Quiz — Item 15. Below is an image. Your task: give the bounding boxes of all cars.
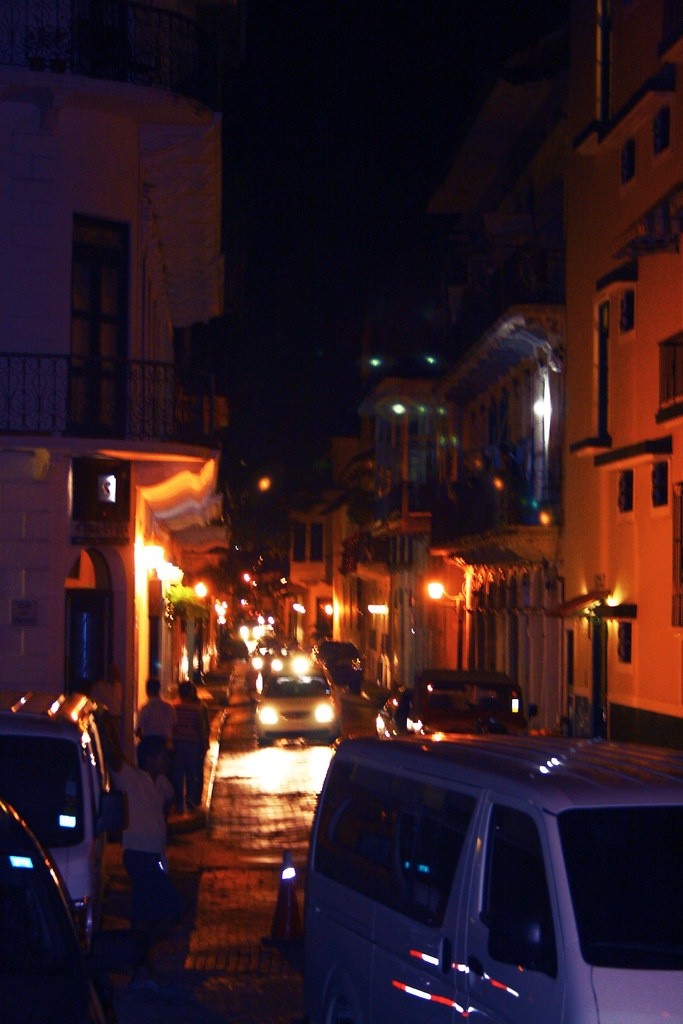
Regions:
[407,668,527,732]
[257,671,340,745]
[313,640,364,693]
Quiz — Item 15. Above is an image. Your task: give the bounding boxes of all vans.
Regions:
[1,691,114,956]
[0,799,120,1024]
[300,730,683,1023]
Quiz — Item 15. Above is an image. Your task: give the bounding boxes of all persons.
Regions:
[141,677,210,814]
[104,737,183,980]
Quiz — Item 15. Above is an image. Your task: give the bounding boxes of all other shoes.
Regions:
[188,801,197,814]
[172,800,184,816]
